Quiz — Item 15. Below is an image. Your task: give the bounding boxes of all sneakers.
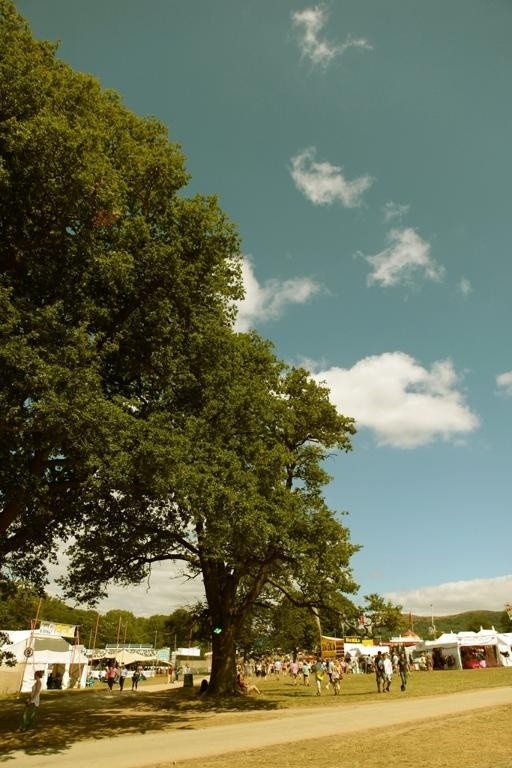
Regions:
[378,684,405,693]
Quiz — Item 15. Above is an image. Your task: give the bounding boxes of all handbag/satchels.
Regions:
[317,673,323,681]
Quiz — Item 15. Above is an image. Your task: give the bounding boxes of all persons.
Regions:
[87,662,191,691]
[53,672,62,688]
[237,651,433,696]
[47,672,52,688]
[16,670,45,732]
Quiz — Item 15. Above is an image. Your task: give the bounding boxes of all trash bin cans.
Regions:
[184,674,193,687]
